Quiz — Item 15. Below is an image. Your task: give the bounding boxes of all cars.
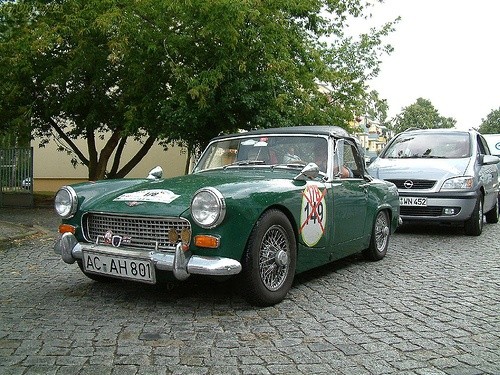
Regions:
[366,127,500,236]
[54,125,401,305]
